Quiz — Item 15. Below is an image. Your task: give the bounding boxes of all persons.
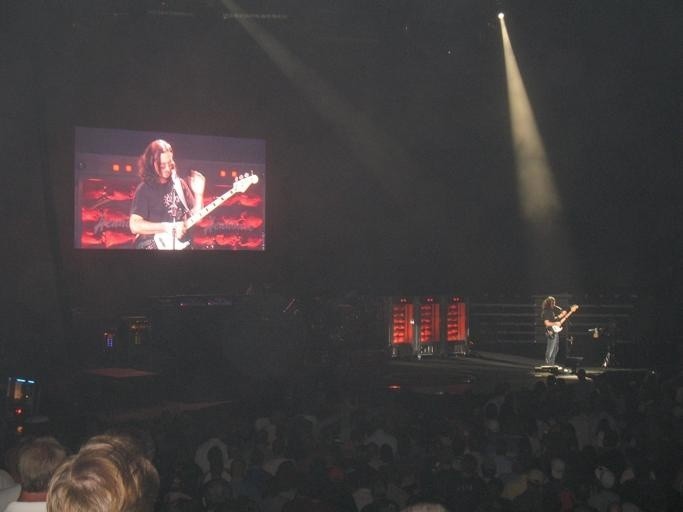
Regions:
[540,295,567,366]
[592,318,618,369]
[128,138,207,251]
[0,365,683,511]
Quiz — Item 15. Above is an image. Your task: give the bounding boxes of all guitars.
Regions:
[543,305,577,339]
[134,170,258,251]
